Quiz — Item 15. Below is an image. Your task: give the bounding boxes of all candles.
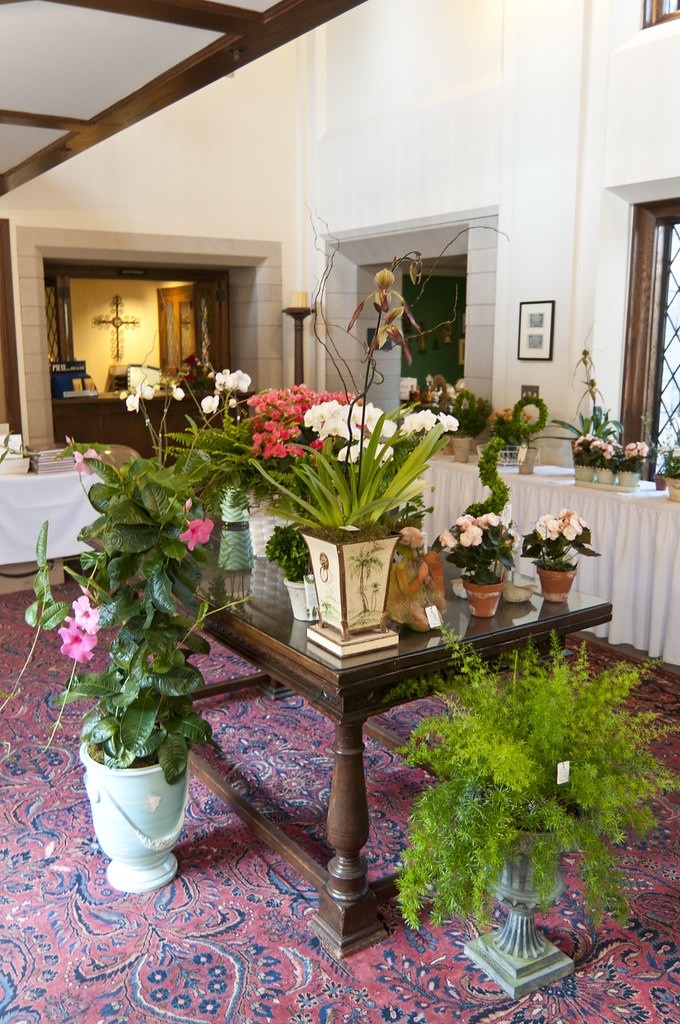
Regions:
[289,290,308,308]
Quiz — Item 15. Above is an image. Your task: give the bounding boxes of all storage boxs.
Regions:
[0,556,65,596]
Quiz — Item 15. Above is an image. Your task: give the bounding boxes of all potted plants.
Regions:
[511,396,548,474]
[264,525,319,622]
[447,390,493,463]
[393,628,680,1001]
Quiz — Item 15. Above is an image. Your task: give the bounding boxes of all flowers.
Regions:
[0,219,680,785]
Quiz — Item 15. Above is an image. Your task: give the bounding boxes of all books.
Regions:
[25,441,77,477]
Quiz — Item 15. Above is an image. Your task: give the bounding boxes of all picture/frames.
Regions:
[518,300,555,361]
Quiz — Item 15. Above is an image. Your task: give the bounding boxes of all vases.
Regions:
[617,471,640,487]
[247,494,294,558]
[537,567,576,603]
[462,577,504,617]
[298,529,404,660]
[219,485,254,570]
[575,465,595,482]
[80,744,189,893]
[595,467,615,485]
[653,474,668,491]
[665,477,680,502]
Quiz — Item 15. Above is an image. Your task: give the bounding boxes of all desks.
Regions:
[420,454,680,668]
[51,388,256,468]
[0,474,105,565]
[84,501,613,961]
